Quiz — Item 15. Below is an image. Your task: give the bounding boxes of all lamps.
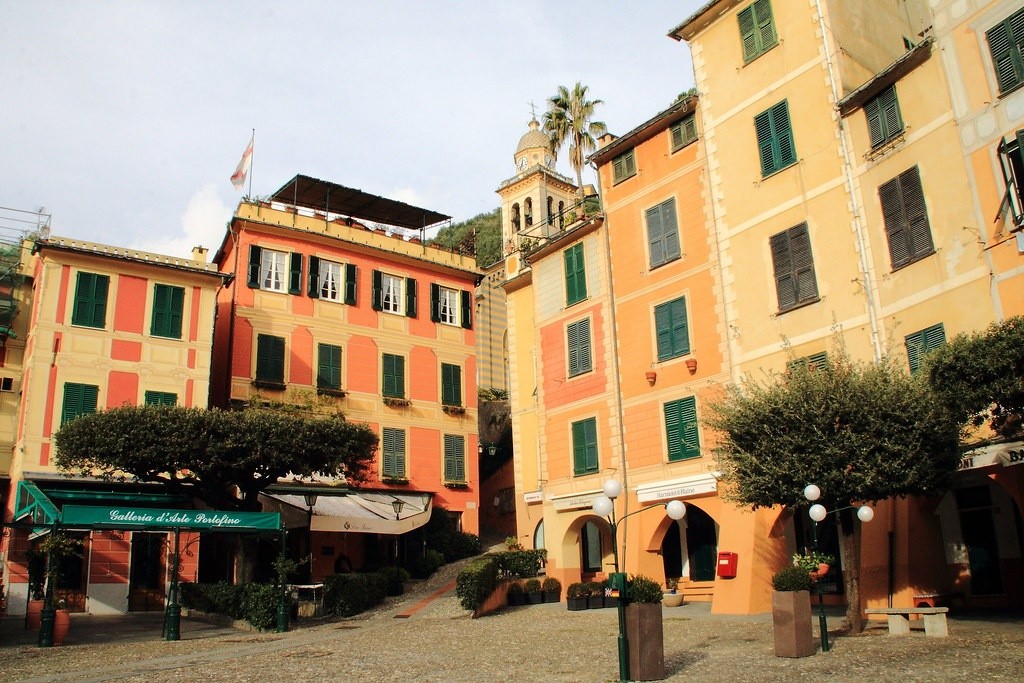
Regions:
[488,442,497,455]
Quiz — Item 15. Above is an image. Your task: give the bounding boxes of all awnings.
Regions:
[262,486,434,536]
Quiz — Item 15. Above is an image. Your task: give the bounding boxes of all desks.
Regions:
[913,593,942,620]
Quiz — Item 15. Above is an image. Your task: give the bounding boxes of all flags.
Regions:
[229,138,253,192]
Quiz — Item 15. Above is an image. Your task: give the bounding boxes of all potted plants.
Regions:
[772,566,815,658]
[23,548,46,634]
[506,577,684,611]
[623,572,666,681]
[40,553,85,646]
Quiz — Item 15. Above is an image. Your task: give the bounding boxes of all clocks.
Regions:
[517,156,528,171]
[543,154,556,169]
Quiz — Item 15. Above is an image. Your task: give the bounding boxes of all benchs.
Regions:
[865,607,949,637]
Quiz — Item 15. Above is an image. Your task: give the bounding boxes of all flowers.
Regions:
[791,552,835,582]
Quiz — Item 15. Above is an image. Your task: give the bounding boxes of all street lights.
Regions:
[804,484,875,653]
[591,480,686,683]
